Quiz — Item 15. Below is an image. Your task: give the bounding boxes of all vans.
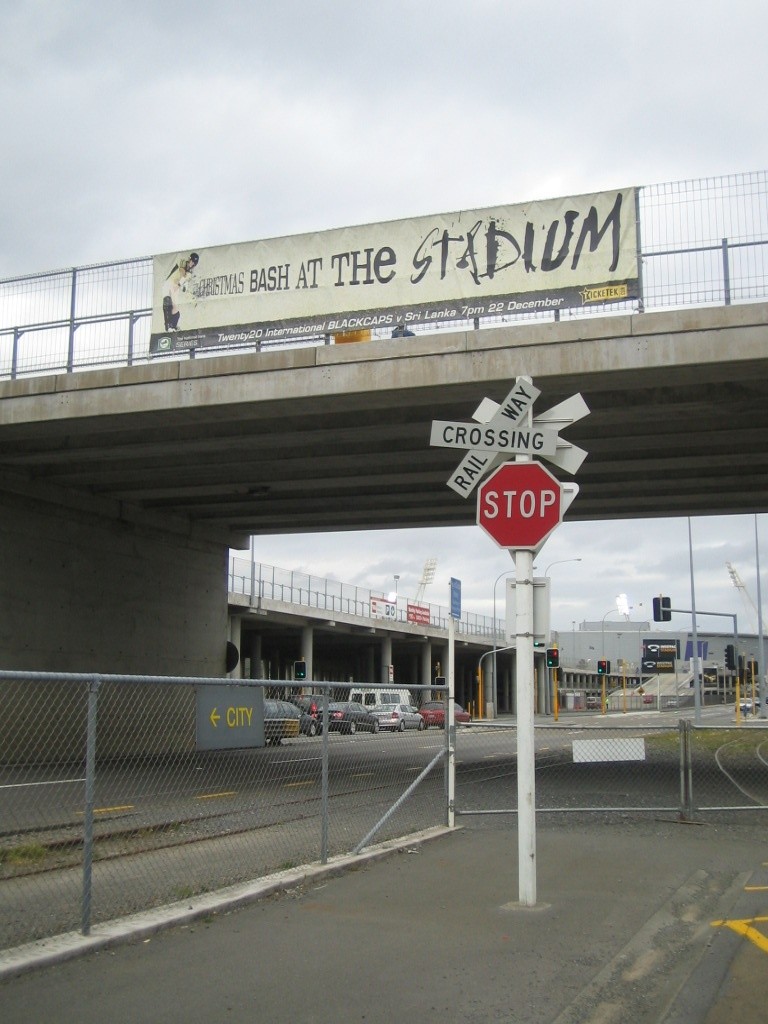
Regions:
[348,687,419,712]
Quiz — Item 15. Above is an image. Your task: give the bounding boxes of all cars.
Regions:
[287,694,380,735]
[419,701,472,729]
[264,698,318,741]
[735,697,760,712]
[369,703,425,732]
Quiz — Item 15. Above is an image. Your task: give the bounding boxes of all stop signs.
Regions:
[475,461,564,549]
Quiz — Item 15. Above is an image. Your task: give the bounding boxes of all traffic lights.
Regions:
[534,642,545,647]
[597,660,607,674]
[546,648,560,668]
[723,644,734,670]
[294,661,307,679]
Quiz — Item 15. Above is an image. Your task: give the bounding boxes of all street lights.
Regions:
[493,567,537,719]
[545,558,583,577]
